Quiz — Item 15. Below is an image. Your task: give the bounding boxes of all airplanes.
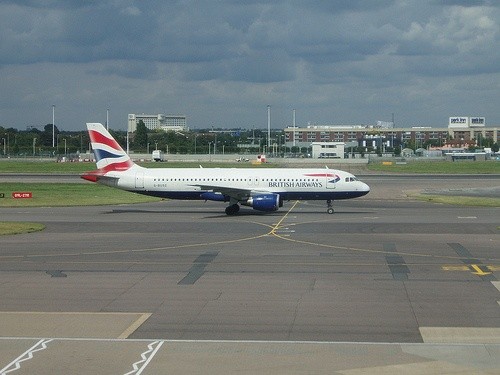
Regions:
[79,121,370,217]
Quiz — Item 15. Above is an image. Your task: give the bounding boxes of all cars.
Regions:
[235,156,249,162]
